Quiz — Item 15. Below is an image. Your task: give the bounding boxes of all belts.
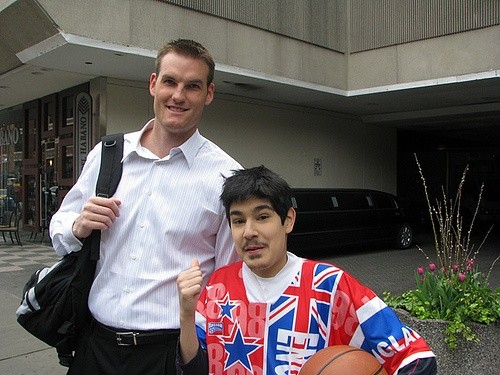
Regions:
[91,317,180,346]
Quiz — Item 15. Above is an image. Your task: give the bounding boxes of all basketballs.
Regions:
[299,345,388,375]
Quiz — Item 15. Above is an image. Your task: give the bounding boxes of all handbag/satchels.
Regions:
[16,248,100,366]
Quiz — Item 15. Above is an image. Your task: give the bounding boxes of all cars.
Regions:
[285,188,426,252]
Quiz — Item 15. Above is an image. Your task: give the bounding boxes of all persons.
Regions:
[48,37,246,375]
[176,166,438,375]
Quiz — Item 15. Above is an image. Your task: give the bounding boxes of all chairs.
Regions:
[0,210,51,246]
